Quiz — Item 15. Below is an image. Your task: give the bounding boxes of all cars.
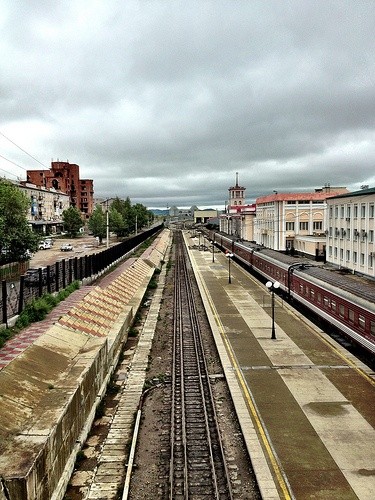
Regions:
[37,241,49,251]
[44,239,53,245]
[60,244,74,252]
[24,266,55,286]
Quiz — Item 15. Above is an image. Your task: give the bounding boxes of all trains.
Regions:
[197,225,375,356]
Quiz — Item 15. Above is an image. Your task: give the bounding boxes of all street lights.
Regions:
[225,253,234,284]
[266,281,280,339]
[197,232,202,251]
[210,240,217,262]
